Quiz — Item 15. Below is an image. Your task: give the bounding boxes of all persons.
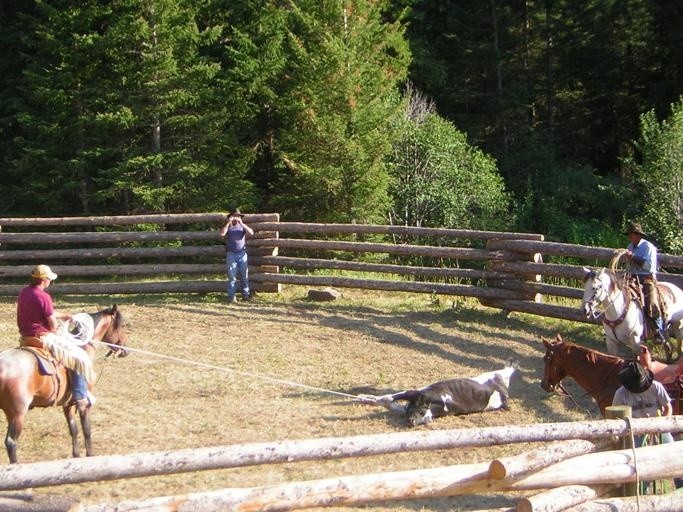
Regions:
[218,207,254,304]
[612,362,683,496]
[17,264,91,412]
[622,223,667,344]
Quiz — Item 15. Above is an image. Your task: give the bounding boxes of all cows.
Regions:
[356,354,520,428]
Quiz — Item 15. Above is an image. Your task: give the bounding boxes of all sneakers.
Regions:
[77,397,95,408]
[228,296,238,302]
[241,293,251,300]
[654,336,665,345]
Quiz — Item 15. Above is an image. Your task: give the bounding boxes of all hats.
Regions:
[228,209,244,218]
[31,264,57,280]
[622,223,647,235]
[618,361,654,394]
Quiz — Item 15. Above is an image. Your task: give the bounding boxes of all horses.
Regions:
[580,265,683,362]
[0,303,130,464]
[540,331,683,420]
